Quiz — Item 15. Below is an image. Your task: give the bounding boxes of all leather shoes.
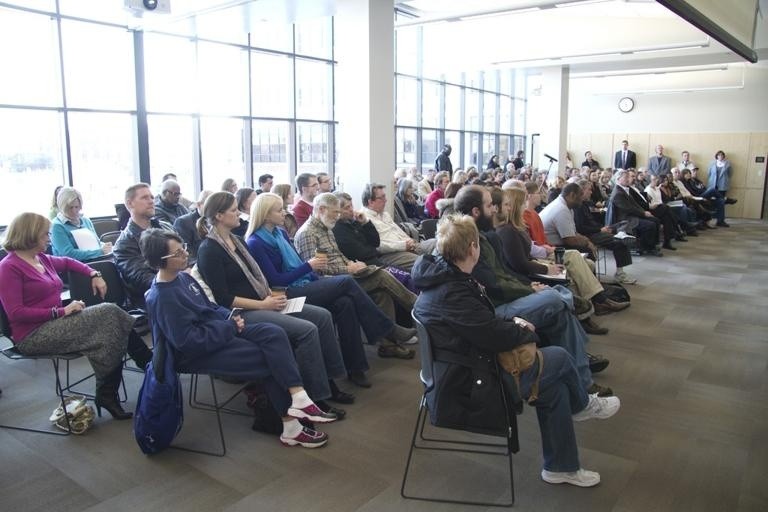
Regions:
[683,222,699,231]
[662,239,676,250]
[329,379,356,404]
[724,197,737,204]
[647,247,663,257]
[316,400,345,421]
[580,318,609,334]
[592,292,630,316]
[717,220,730,227]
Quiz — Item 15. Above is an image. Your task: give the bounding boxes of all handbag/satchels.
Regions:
[50,396,96,435]
[244,377,292,432]
[498,321,543,405]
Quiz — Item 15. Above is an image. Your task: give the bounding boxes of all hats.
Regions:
[688,163,699,171]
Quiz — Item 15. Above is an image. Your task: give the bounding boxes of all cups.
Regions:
[270,286,286,309]
[554,247,565,263]
[315,248,328,270]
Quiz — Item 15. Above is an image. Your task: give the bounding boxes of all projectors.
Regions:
[123,0,170,15]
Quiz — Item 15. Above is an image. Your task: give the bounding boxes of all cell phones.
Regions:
[227,308,242,320]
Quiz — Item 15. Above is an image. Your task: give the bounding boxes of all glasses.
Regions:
[160,243,187,259]
[320,179,331,183]
[324,206,341,216]
[166,190,182,197]
[529,192,541,195]
[602,177,609,178]
[43,232,52,238]
[375,194,386,200]
[303,183,319,187]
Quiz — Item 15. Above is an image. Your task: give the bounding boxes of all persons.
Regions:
[394,168,407,181]
[397,178,419,220]
[418,169,436,199]
[676,152,695,171]
[574,180,637,284]
[507,189,571,289]
[522,182,596,275]
[453,163,548,205]
[140,229,337,448]
[502,180,630,335]
[615,140,636,169]
[162,174,195,208]
[393,178,418,229]
[173,190,218,255]
[503,155,515,172]
[0,212,154,419]
[331,191,383,267]
[486,187,609,374]
[581,151,599,171]
[436,183,463,217]
[408,168,419,179]
[453,185,610,396]
[295,193,418,360]
[487,155,500,169]
[273,184,298,238]
[604,168,738,257]
[154,180,191,229]
[547,166,617,212]
[513,151,524,170]
[411,212,621,488]
[359,185,437,272]
[50,187,112,261]
[566,152,573,169]
[707,150,732,195]
[293,173,320,228]
[538,184,597,262]
[49,186,62,220]
[317,172,331,192]
[236,189,258,235]
[256,174,273,194]
[424,171,450,218]
[434,145,452,181]
[112,182,173,306]
[244,193,417,387]
[222,178,238,193]
[196,192,355,422]
[646,145,672,176]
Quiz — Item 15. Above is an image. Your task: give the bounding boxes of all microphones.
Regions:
[543,154,558,163]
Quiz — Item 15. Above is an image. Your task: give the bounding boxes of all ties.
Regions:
[685,161,687,167]
[622,152,625,170]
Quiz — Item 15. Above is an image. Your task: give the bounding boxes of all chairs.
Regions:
[401,308,513,508]
[93,220,121,246]
[143,290,257,457]
[0,301,127,436]
[65,261,147,403]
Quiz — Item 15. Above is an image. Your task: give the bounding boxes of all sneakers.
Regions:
[572,395,620,422]
[587,382,613,397]
[378,344,416,360]
[396,336,418,344]
[613,272,637,285]
[586,351,609,374]
[541,469,600,487]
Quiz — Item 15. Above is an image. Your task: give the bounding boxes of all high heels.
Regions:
[674,225,689,242]
[135,344,152,373]
[95,385,133,420]
[385,324,417,345]
[347,370,371,388]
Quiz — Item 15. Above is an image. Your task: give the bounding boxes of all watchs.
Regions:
[90,272,103,277]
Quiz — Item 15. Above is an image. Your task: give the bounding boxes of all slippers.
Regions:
[280,426,329,448]
[287,404,338,424]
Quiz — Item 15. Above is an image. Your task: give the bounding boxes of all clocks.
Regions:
[618,97,634,113]
